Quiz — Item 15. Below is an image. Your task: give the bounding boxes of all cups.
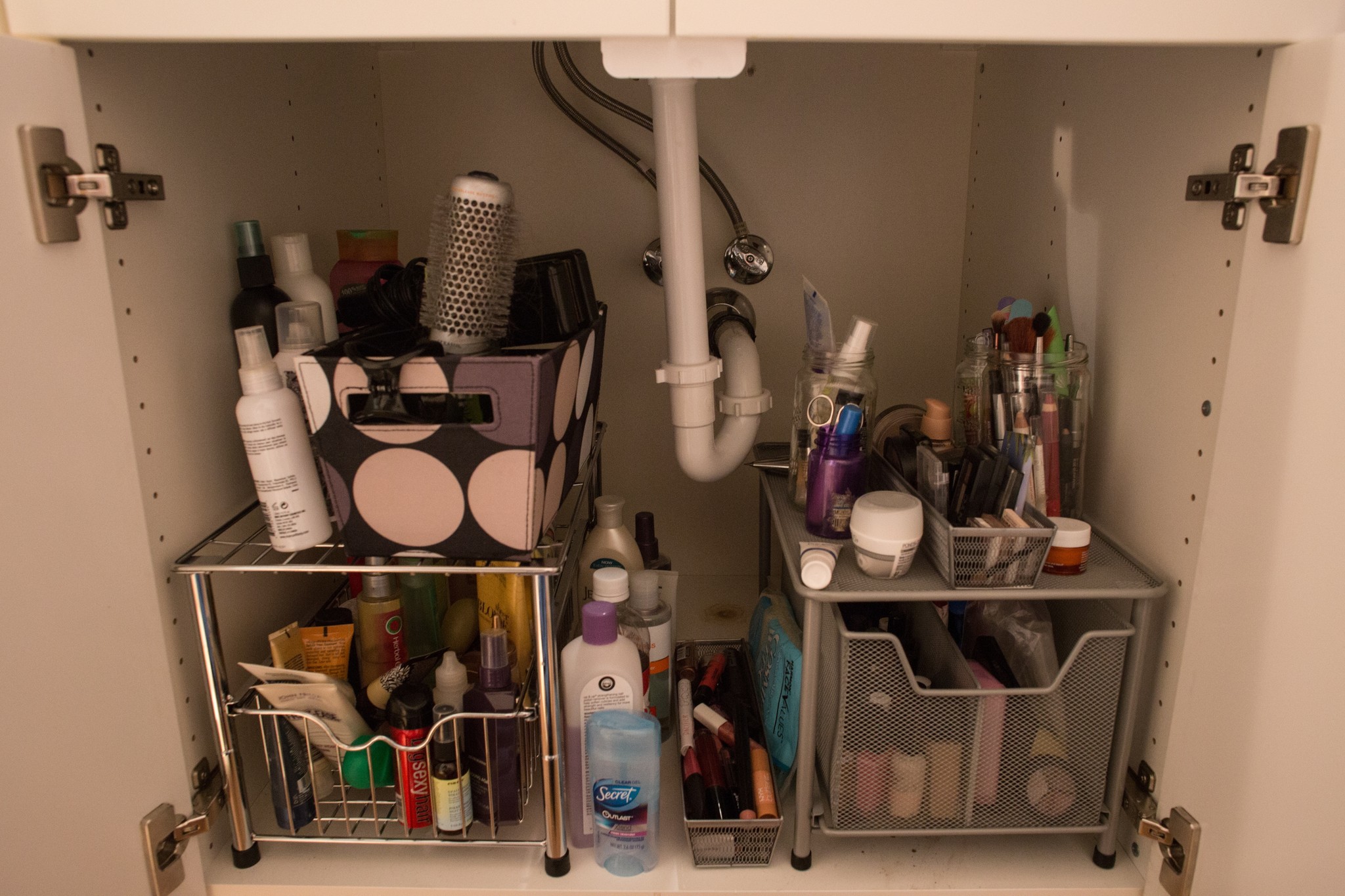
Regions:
[794,341,877,516]
[978,340,1090,523]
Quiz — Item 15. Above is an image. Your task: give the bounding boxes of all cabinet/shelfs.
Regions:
[0,0,1345,894]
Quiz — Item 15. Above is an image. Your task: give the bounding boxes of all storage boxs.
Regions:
[296,299,610,562]
